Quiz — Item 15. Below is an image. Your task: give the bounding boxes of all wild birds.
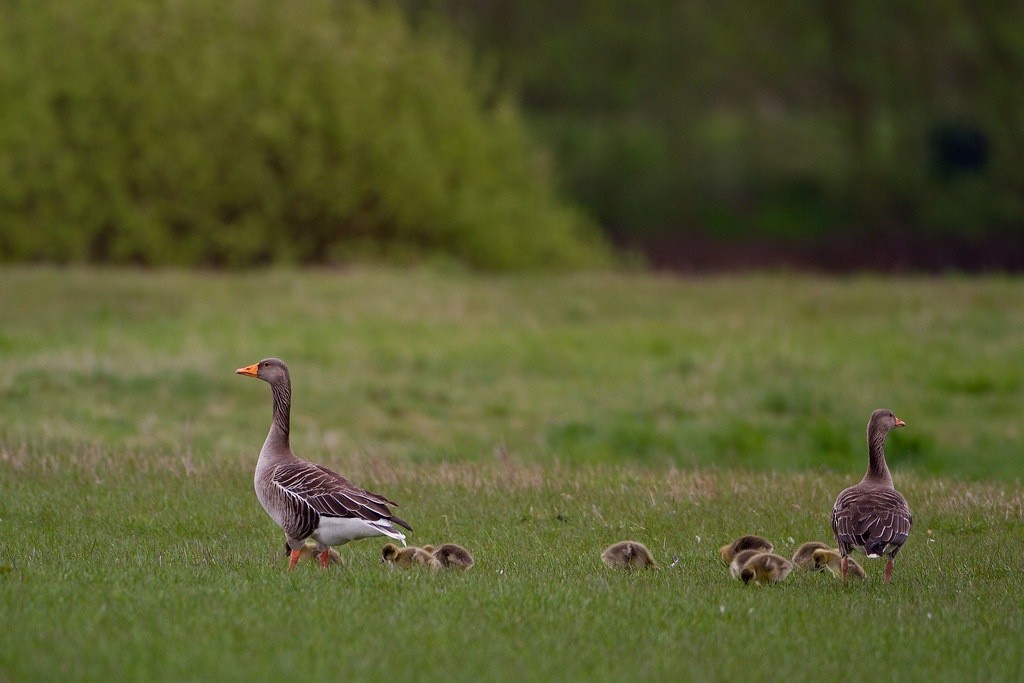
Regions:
[237,358,475,574]
[718,534,866,588]
[830,408,913,591]
[600,541,659,585]
